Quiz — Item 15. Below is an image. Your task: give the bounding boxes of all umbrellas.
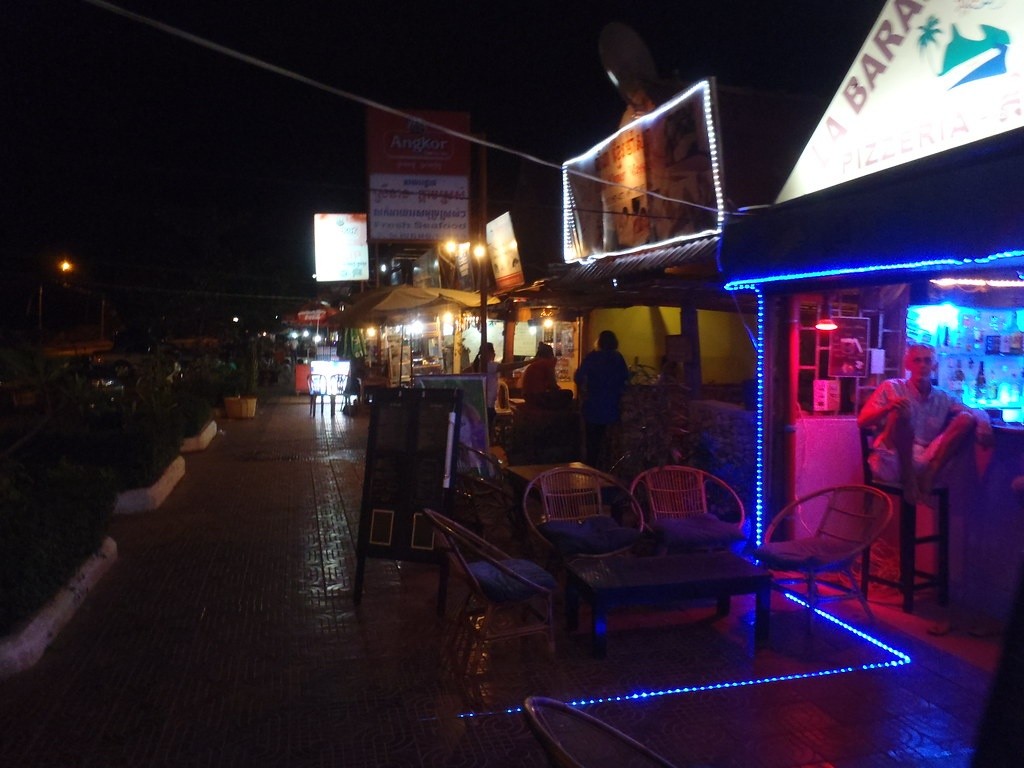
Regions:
[324,284,500,388]
[281,301,341,337]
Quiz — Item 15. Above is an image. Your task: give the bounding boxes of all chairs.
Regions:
[522,467,646,571]
[521,696,677,768]
[752,485,894,637]
[306,374,360,418]
[424,509,559,680]
[629,466,746,554]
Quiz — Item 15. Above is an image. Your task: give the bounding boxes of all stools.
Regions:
[860,481,951,613]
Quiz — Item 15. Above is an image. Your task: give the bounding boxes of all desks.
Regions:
[565,547,773,661]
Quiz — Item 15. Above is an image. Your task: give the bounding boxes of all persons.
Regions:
[522,341,573,407]
[613,208,650,239]
[461,342,533,436]
[574,330,633,469]
[855,343,994,510]
[458,409,487,474]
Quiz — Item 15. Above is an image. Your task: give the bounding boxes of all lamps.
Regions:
[814,285,838,331]
